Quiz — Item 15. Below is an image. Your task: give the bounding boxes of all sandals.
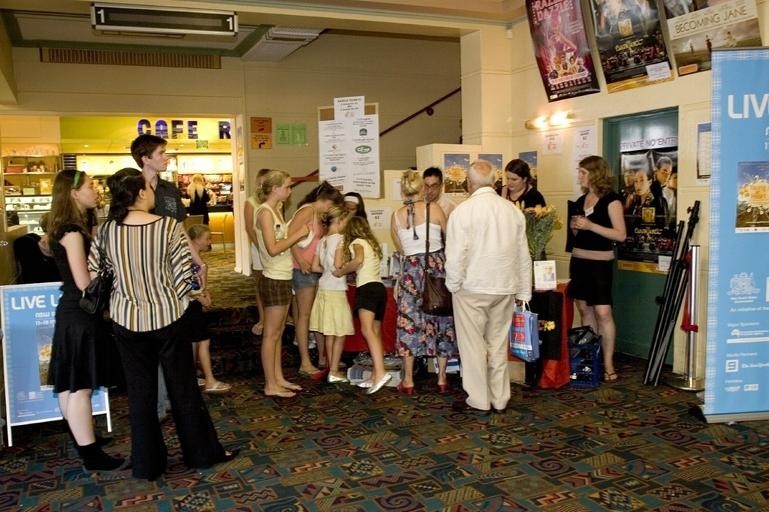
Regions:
[604,370,619,381]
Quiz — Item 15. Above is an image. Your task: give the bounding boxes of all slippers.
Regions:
[263,382,304,399]
[197,378,232,394]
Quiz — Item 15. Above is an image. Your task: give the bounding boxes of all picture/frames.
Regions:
[696,122,712,186]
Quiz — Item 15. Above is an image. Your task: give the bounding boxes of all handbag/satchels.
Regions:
[78,272,112,316]
[422,271,455,317]
[508,297,542,365]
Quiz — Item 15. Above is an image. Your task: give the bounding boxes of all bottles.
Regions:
[274,224,285,255]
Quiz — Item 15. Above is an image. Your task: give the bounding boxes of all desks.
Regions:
[344,280,574,389]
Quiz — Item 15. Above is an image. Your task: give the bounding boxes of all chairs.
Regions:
[210,215,228,255]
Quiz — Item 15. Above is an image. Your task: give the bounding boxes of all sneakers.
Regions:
[251,316,317,350]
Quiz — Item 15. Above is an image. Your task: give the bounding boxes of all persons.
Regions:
[187,174,211,226]
[129,134,187,426]
[423,167,456,218]
[566,155,628,382]
[39,169,127,473]
[620,156,677,215]
[85,168,239,480]
[444,159,534,414]
[187,223,232,392]
[389,169,458,395]
[494,158,547,262]
[244,167,393,399]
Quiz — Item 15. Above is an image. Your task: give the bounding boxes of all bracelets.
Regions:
[200,263,209,267]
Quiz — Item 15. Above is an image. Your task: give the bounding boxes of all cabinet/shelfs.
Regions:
[4,171,56,212]
[178,173,233,205]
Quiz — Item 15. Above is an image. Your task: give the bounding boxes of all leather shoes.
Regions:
[451,398,508,415]
[357,372,392,396]
[396,382,414,397]
[220,446,243,461]
[296,360,351,386]
[437,383,451,394]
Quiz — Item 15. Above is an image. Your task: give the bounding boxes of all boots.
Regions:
[71,440,129,475]
